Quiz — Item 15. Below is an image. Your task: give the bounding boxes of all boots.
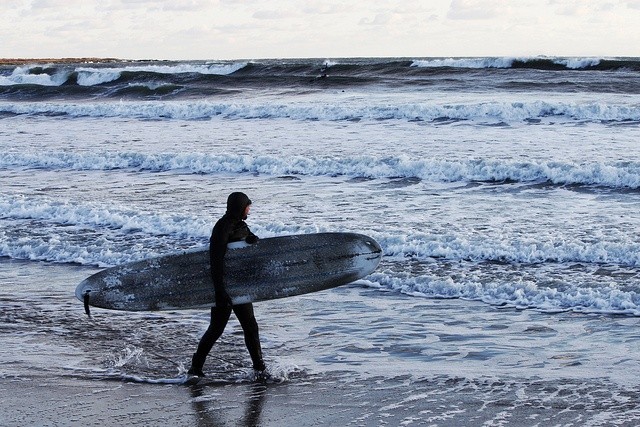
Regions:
[187,352,206,376]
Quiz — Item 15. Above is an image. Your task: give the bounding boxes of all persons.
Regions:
[183,192,274,381]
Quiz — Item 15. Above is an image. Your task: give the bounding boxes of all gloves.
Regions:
[245,235,258,244]
[215,289,233,310]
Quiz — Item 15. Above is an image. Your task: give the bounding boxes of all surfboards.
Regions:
[75,232,383,312]
